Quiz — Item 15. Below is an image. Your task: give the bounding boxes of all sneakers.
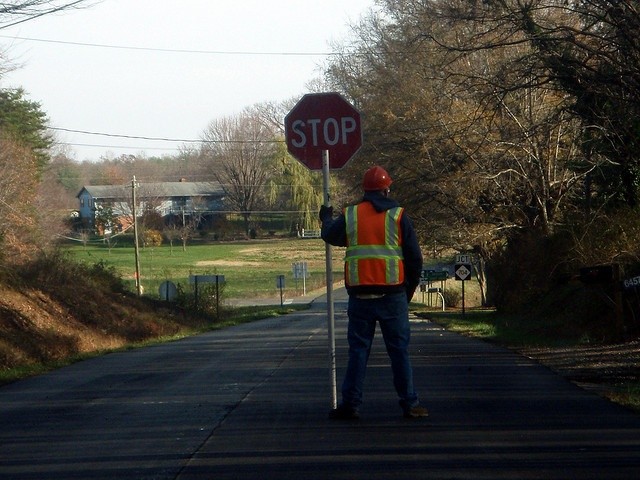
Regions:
[329,407,359,419]
[403,405,428,417]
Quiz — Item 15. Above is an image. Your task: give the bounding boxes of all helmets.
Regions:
[364,166,392,190]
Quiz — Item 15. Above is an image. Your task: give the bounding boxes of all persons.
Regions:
[319,165,428,419]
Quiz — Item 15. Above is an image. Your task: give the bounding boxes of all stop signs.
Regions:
[287,89,361,172]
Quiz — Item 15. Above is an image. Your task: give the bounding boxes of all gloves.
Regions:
[319,205,333,221]
